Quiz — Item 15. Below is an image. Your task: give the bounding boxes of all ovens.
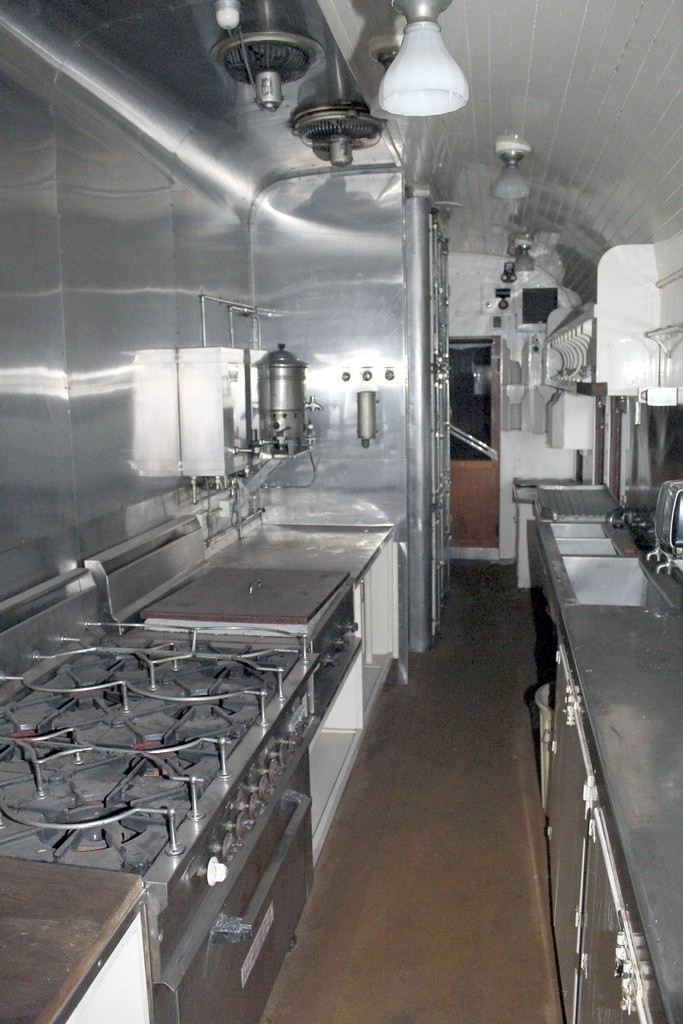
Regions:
[155,744,312,1024]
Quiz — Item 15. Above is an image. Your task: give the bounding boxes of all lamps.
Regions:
[379,0,469,116]
[513,239,534,271]
[494,132,531,199]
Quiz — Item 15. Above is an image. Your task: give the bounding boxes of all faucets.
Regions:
[654,546,675,578]
[646,535,663,564]
[605,504,627,523]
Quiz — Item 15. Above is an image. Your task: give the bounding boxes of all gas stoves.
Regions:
[0,571,321,981]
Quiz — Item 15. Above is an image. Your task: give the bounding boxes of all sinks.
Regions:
[547,520,609,539]
[559,556,676,609]
[553,539,623,557]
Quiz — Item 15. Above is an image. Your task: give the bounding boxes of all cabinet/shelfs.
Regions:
[544,231,682,406]
[56,531,398,1024]
[547,645,683,1024]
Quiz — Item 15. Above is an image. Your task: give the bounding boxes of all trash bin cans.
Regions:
[513,476,578,590]
[533,682,556,810]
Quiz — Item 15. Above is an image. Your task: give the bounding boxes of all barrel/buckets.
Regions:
[536,682,556,815]
